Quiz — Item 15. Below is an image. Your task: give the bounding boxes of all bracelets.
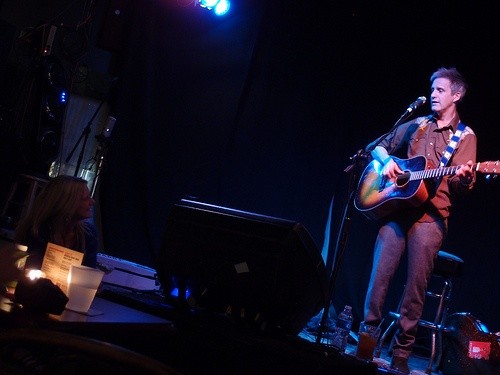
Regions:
[469,185,472,187]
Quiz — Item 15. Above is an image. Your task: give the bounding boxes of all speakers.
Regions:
[157,198,332,337]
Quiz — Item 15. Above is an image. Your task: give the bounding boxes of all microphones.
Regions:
[95,116,117,143]
[393,96,427,127]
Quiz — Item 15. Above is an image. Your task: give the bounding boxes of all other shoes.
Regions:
[391,357,410,375]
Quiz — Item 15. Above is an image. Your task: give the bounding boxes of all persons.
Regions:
[347,67,477,375]
[15,175,98,269]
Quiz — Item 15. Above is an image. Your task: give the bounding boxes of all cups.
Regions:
[355,323,382,361]
[66,264,104,312]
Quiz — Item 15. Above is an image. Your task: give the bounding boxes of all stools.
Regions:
[0,173,50,241]
[373,250,464,374]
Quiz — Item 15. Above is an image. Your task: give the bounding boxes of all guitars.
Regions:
[353,155,500,221]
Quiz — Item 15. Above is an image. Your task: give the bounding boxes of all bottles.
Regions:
[333,304,353,353]
